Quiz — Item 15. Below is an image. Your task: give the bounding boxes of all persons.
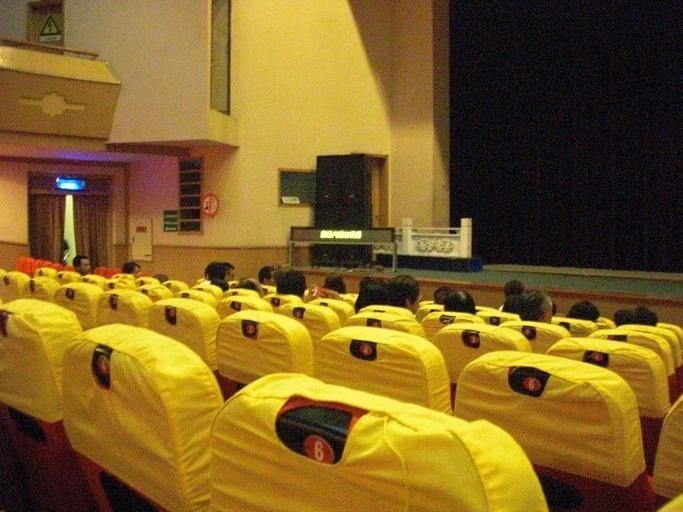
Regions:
[204,262,657,326]
[153,273,168,283]
[62,240,71,263]
[122,263,142,279]
[72,255,91,274]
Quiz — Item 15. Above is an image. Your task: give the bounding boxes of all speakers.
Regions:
[314,154,372,266]
